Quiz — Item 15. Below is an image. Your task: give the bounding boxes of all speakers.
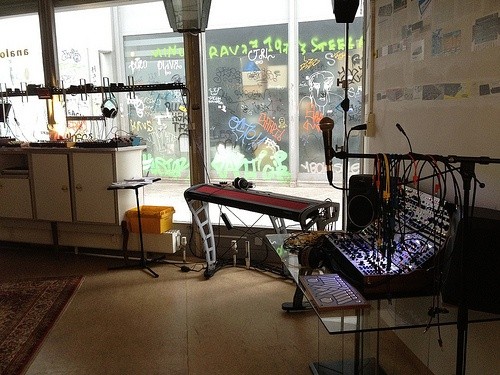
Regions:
[346,174,401,233]
[441,207,500,315]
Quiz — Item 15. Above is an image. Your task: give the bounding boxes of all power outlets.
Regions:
[363,114,375,137]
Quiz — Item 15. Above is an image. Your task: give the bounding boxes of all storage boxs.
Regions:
[125,205,175,234]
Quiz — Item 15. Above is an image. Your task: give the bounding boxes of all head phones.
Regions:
[232,177,253,190]
[101,97,118,118]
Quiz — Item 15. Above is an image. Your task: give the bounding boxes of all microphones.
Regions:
[319,117,335,183]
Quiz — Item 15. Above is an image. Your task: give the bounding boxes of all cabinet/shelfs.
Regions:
[28,149,144,226]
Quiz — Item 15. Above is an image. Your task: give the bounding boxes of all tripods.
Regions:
[109,177,166,279]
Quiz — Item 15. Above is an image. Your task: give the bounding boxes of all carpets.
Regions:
[0,274,86,375]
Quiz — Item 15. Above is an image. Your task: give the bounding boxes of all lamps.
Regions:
[163,0,211,33]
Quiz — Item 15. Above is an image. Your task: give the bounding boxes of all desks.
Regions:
[107,177,161,278]
[264,235,500,375]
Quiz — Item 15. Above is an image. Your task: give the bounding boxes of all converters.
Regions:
[221,213,233,230]
